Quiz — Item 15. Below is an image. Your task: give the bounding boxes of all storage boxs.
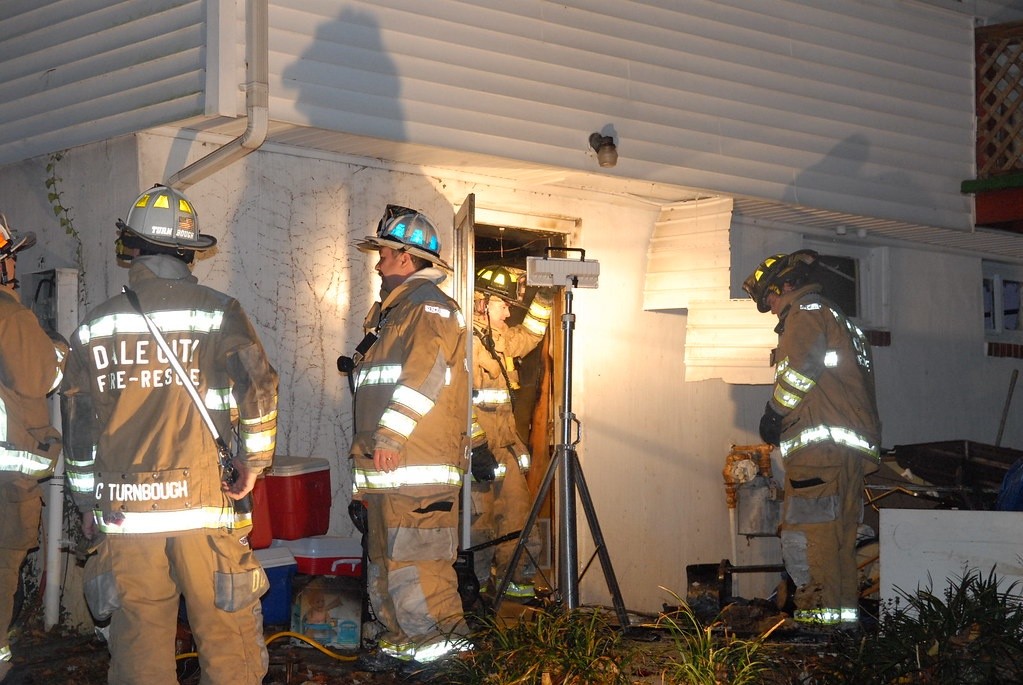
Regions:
[268,457,330,540]
[248,476,273,550]
[281,538,364,576]
[254,547,297,641]
[292,576,361,649]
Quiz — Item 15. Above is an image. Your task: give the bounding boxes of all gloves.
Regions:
[759,400,784,447]
[471,441,499,483]
[539,285,566,300]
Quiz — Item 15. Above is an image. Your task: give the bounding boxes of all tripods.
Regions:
[473,291,633,648]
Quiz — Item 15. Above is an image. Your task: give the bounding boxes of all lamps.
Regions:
[588,134,618,167]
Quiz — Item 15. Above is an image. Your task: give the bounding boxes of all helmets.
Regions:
[474,265,519,302]
[0,213,27,262]
[365,204,453,271]
[114,183,217,250]
[742,248,819,313]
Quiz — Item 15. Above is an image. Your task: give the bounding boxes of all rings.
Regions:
[386,457,392,460]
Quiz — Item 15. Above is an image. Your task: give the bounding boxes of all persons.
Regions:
[58,186,279,685]
[471,264,566,610]
[741,248,883,638]
[0,213,70,683]
[346,212,475,663]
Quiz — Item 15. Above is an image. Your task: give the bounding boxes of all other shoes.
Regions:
[356,647,412,672]
[396,657,426,680]
[767,624,838,644]
[0,667,35,685]
[485,586,547,608]
